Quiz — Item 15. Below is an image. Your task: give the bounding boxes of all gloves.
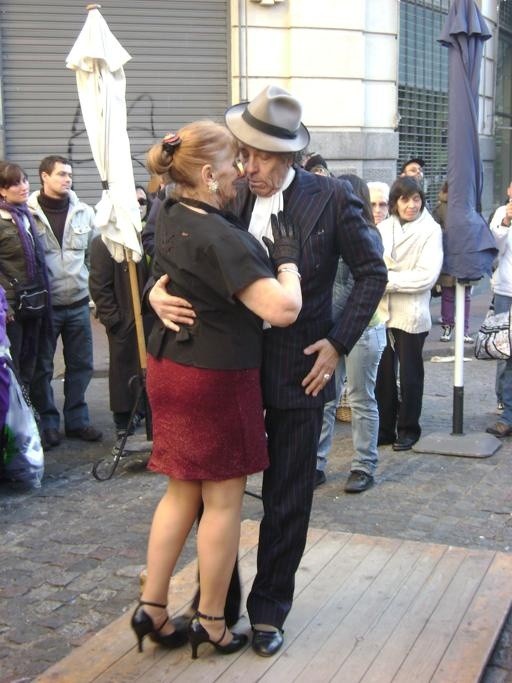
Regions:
[262,210,301,269]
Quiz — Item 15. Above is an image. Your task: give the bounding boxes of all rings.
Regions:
[323,372,330,380]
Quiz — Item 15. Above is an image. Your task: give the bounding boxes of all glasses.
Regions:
[137,199,149,205]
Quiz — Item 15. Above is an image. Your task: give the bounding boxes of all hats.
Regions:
[401,158,424,173]
[224,85,310,153]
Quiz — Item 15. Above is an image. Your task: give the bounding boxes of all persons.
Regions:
[432,181,475,343]
[400,158,425,182]
[376,175,443,450]
[366,181,390,223]
[27,156,101,445]
[131,117,304,656]
[299,151,387,492]
[87,187,145,437]
[1,161,52,450]
[486,178,511,436]
[141,85,386,657]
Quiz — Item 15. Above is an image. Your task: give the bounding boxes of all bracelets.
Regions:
[275,267,302,278]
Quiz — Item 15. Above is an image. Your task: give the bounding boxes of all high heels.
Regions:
[190,618,248,659]
[132,602,188,652]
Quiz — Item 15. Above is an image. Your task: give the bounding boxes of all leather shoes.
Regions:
[251,624,284,657]
[173,608,237,629]
[392,439,416,450]
[343,470,374,491]
[313,470,325,488]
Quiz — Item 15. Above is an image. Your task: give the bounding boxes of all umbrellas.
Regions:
[437,1,502,436]
[66,4,154,439]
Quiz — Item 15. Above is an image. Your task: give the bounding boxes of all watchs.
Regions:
[141,85,386,657]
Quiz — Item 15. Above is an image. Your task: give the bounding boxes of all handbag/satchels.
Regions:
[15,289,50,321]
[474,309,511,360]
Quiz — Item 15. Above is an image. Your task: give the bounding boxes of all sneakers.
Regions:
[112,434,134,457]
[463,336,474,343]
[486,421,512,437]
[42,428,60,446]
[440,325,452,342]
[66,427,104,441]
